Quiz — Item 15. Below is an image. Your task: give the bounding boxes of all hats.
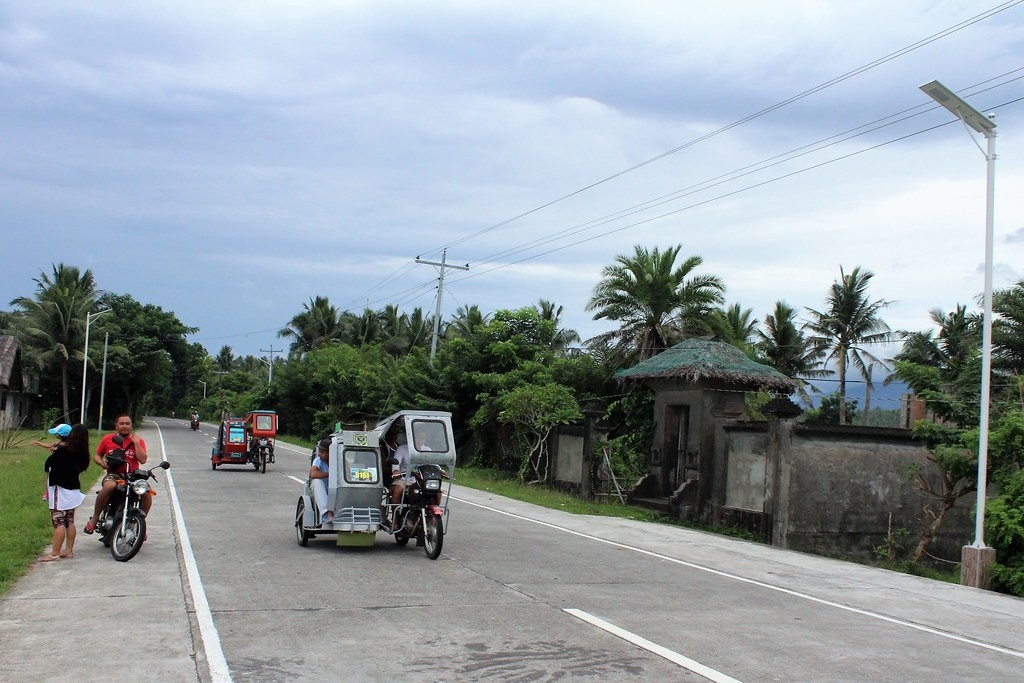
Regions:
[317,439,332,450]
[48,424,72,436]
[195,411,198,413]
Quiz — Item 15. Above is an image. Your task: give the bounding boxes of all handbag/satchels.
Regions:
[105,448,126,469]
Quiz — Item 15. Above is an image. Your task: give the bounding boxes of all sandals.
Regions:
[37,555,60,562]
[59,552,74,558]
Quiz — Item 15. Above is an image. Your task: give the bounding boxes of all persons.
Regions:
[83,416,157,542]
[249,417,274,464]
[30,423,91,562]
[190,411,200,429]
[390,427,449,531]
[309,439,353,525]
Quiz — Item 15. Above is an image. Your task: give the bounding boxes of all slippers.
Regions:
[325,516,333,524]
[84,522,95,534]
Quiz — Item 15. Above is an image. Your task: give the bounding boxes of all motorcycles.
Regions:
[295,410,456,559]
[190,418,199,432]
[94,436,170,562]
[211,410,279,473]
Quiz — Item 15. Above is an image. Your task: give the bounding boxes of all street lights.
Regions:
[80,309,114,424]
[917,79,997,590]
[198,379,206,399]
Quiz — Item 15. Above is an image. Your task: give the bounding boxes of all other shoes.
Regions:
[43,492,49,501]
